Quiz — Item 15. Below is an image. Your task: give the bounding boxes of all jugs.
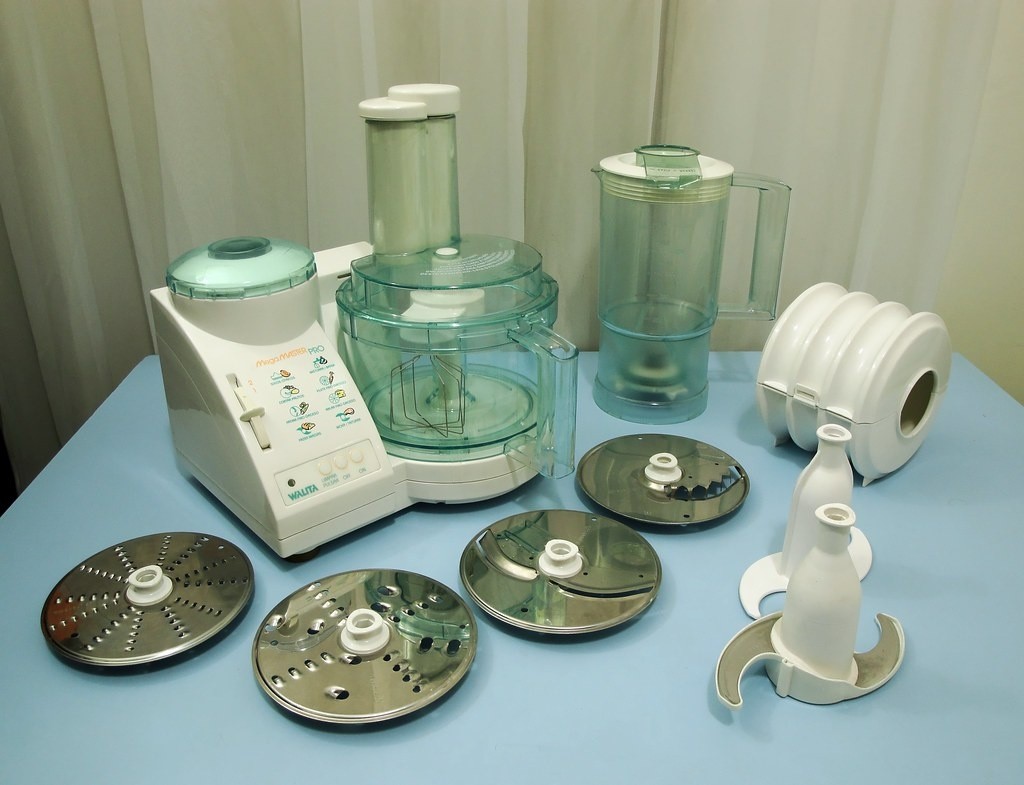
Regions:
[591,145,791,425]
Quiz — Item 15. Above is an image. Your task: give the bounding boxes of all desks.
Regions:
[0,347,1024,785]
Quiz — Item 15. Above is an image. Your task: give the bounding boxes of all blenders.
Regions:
[148,84,578,559]
